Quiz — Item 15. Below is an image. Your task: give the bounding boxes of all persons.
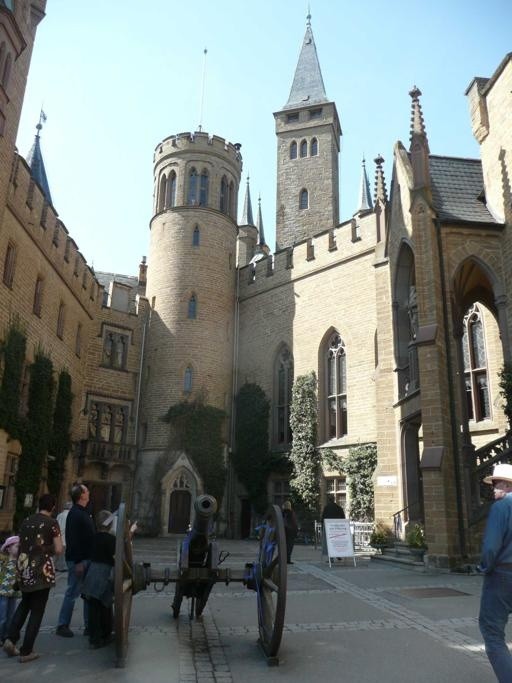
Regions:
[0,482,138,664]
[321,494,345,563]
[281,501,298,564]
[475,464,512,682]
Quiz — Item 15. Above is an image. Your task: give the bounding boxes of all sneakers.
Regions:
[4,639,20,656]
[21,652,40,663]
[56,625,73,637]
[84,628,114,648]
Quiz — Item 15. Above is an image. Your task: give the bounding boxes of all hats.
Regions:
[1,536,20,553]
[483,463,512,484]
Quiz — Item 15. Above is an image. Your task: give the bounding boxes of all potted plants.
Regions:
[403,521,429,564]
[368,522,391,557]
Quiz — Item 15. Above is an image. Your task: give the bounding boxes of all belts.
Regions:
[496,563,512,569]
